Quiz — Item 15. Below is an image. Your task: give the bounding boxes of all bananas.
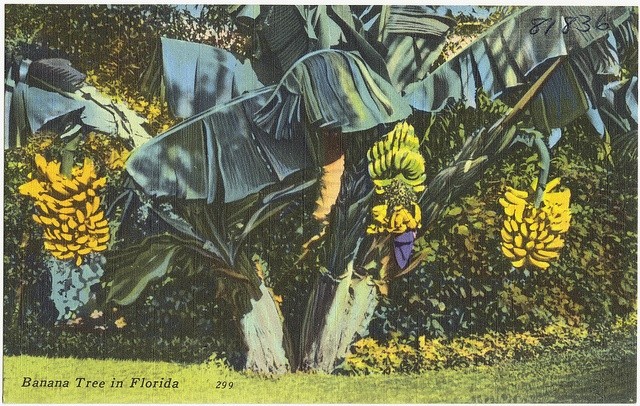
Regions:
[499,176,572,270]
[365,121,427,235]
[18,152,110,266]
